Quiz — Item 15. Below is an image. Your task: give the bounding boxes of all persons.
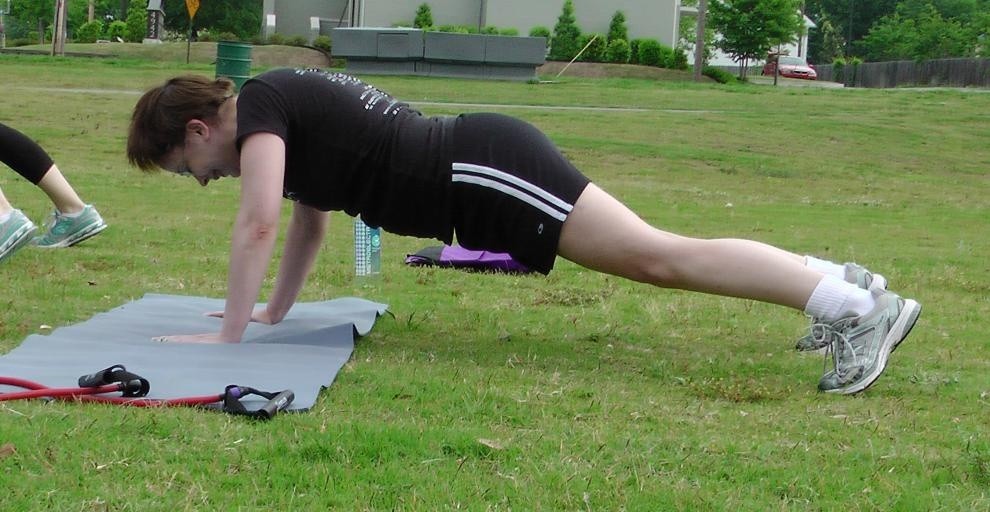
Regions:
[0,122,108,259]
[123,66,924,396]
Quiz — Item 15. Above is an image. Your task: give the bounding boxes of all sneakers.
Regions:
[1,207,39,260]
[818,287,921,396]
[796,261,887,352]
[32,205,106,252]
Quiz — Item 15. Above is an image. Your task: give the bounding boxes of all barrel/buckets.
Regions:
[214,38,253,94]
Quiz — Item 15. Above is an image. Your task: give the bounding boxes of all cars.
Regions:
[761,50,817,82]
